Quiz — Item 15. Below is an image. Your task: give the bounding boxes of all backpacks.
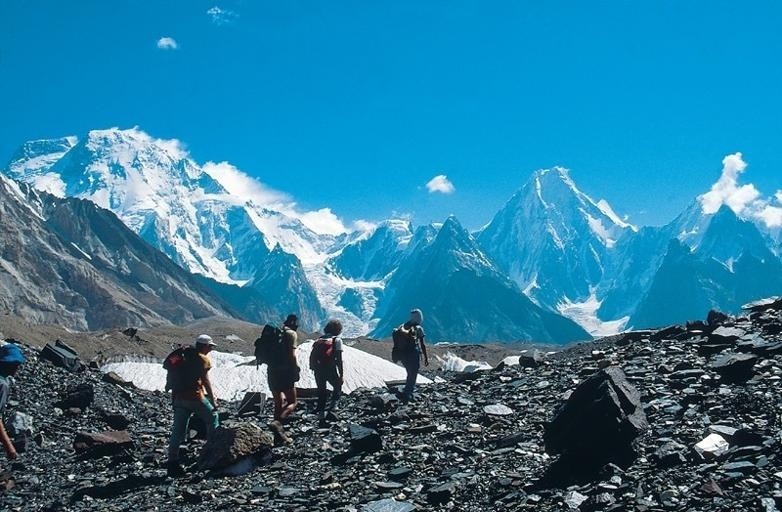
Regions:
[254,321,293,363]
[309,336,338,371]
[395,323,420,350]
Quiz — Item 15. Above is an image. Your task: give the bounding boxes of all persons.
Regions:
[169,334,219,463]
[0,344,27,459]
[266,314,344,442]
[401,308,429,400]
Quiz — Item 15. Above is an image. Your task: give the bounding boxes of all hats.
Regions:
[410,309,423,321]
[0,343,26,365]
[196,334,218,347]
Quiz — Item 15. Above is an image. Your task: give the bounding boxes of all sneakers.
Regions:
[270,421,294,445]
